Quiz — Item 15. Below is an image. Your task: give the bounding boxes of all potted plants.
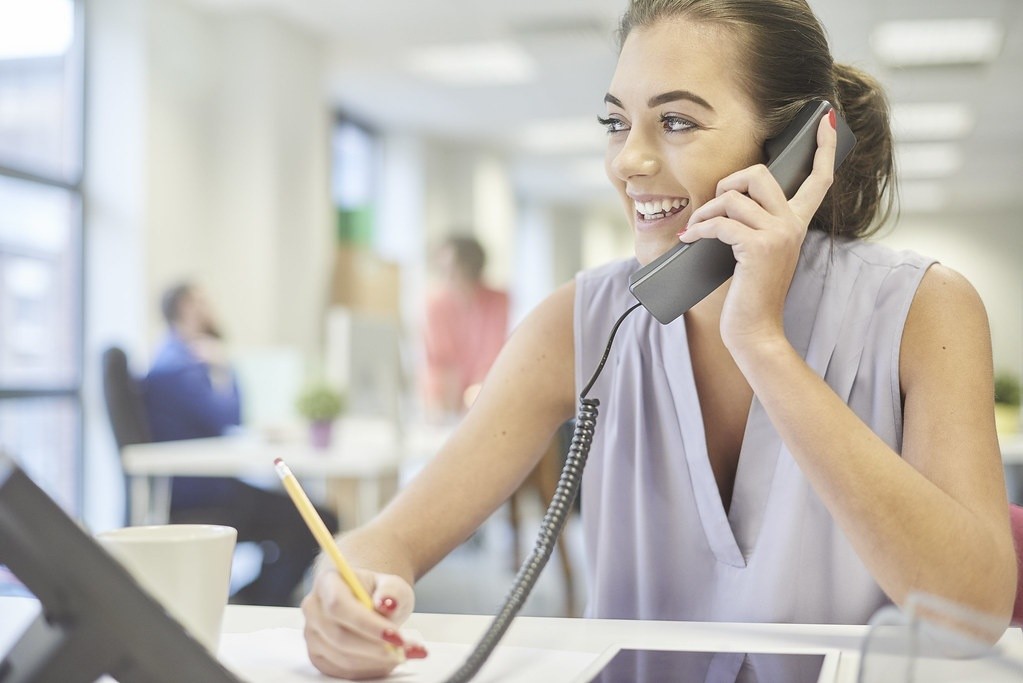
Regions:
[292,374,345,456]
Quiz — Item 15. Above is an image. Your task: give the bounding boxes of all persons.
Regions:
[136,278,340,606]
[421,236,510,413]
[301,0,1016,681]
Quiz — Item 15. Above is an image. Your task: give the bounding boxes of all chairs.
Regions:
[96,343,283,566]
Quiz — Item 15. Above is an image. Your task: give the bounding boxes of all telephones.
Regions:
[629,99,858,325]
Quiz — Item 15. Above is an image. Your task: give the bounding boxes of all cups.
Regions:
[93,524,238,662]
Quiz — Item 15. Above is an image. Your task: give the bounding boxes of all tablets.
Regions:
[572,640,838,682]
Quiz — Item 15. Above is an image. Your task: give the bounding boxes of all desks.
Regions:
[117,410,451,506]
[0,590,1023,683]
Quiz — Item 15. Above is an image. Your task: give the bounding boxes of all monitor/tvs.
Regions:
[1,448,247,683]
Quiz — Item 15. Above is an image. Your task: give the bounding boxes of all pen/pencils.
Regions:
[273,457,406,662]
